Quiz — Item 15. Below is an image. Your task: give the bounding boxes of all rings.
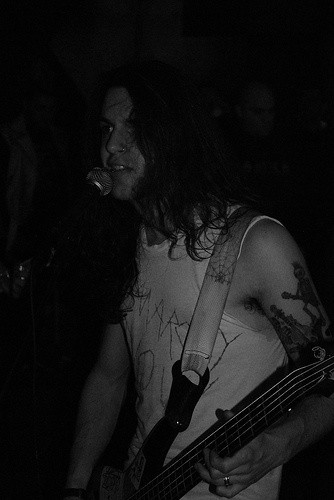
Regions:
[222,475,233,488]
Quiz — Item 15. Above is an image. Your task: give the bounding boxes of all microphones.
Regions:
[80,167,115,211]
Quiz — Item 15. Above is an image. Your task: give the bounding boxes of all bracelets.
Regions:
[59,487,88,499]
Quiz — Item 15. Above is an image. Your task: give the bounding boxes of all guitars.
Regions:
[82,342,334,500]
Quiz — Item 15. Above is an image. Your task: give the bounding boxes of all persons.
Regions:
[55,71,334,499]
